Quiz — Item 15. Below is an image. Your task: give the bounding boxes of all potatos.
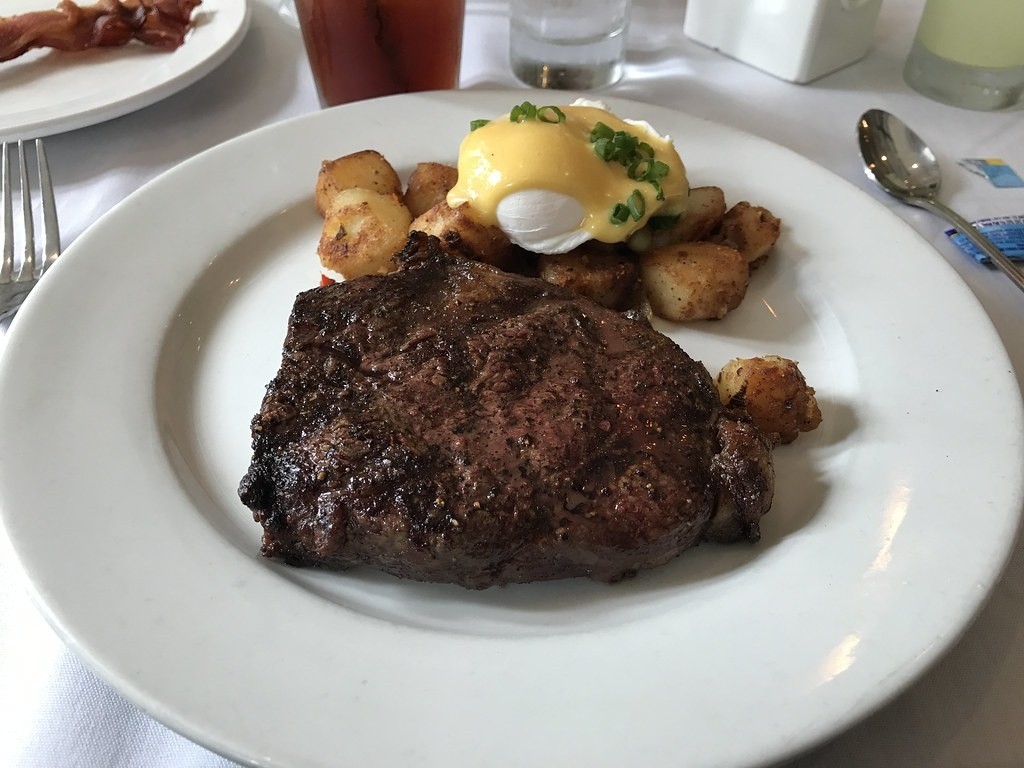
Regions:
[641,186,780,321]
[316,150,512,278]
[719,356,824,446]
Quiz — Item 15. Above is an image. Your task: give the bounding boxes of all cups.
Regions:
[904,0,1024,111]
[293,0,466,109]
[509,0,630,89]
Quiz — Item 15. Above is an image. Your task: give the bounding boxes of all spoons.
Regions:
[856,108,1023,294]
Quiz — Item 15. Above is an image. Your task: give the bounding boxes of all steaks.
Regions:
[238,231,774,588]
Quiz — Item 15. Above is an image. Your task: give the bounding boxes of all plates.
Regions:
[0,0,252,144]
[0,89,1023,768]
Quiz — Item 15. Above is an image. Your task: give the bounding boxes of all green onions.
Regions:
[470,101,670,224]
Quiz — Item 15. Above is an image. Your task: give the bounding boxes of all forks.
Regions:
[0,137,61,319]
